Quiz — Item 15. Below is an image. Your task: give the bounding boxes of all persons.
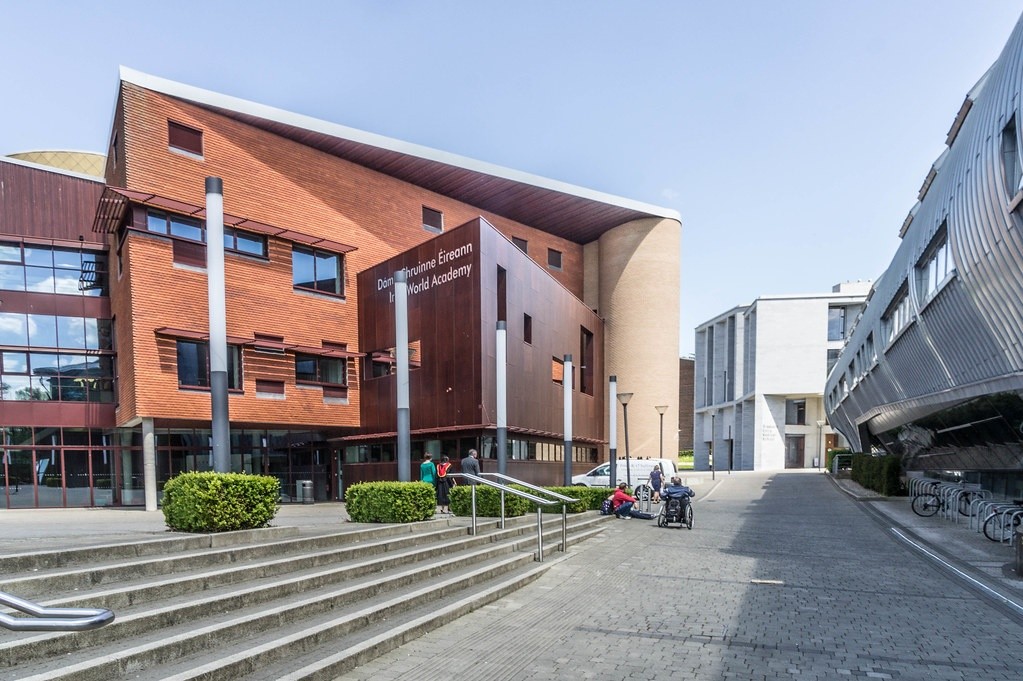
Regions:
[420,452,437,487]
[645,465,665,503]
[461,449,481,486]
[668,478,695,517]
[436,456,457,514]
[601,483,638,519]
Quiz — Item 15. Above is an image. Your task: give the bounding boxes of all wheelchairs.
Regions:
[656,491,694,530]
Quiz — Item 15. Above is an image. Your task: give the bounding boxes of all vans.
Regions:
[571,455,680,501]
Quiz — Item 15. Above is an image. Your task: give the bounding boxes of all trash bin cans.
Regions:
[302,481,315,504]
[813,458,818,468]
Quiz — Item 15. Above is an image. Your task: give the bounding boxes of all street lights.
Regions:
[815,420,826,471]
[706,407,720,480]
[615,393,634,489]
[654,405,669,459]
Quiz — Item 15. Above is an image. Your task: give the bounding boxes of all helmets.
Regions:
[674,478,681,485]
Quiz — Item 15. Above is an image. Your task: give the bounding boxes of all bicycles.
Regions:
[982,498,1023,542]
[910,473,985,518]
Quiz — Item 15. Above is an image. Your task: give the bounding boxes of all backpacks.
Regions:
[600,498,614,514]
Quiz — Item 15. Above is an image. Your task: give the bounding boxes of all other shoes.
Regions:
[625,516,631,519]
[657,502,660,503]
[619,514,625,518]
[653,501,657,503]
